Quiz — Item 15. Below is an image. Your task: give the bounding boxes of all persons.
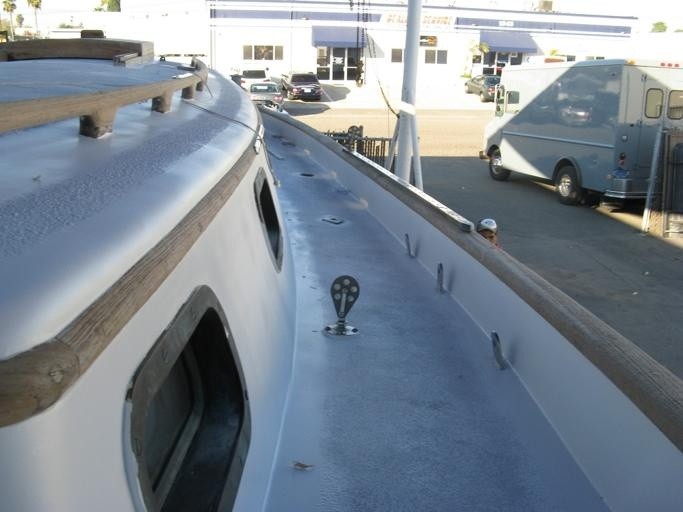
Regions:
[476,217,502,251]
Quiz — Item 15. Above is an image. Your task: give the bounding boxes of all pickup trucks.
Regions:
[237,68,271,92]
[248,82,284,110]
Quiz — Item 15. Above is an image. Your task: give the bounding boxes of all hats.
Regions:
[477,218,497,234]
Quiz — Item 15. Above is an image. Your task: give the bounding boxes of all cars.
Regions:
[465,74,500,102]
[281,72,323,100]
[482,59,683,205]
